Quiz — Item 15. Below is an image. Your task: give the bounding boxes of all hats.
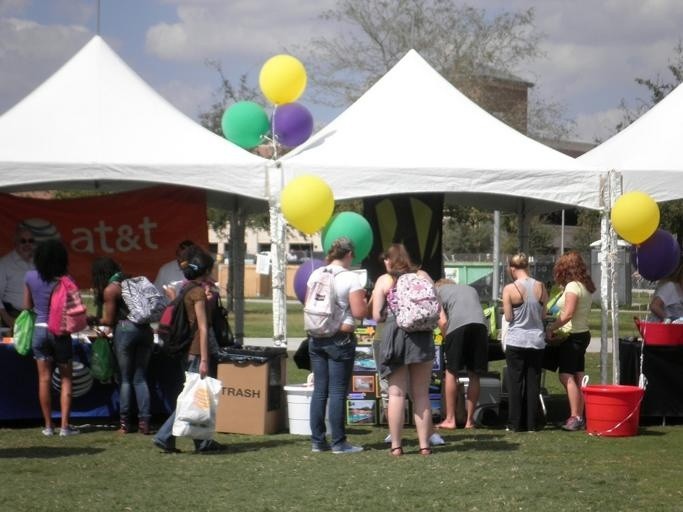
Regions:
[331,236,357,258]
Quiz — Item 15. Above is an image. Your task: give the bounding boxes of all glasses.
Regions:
[17,236,35,245]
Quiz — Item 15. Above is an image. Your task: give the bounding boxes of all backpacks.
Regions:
[385,273,441,333]
[160,283,203,359]
[48,277,88,338]
[304,265,348,338]
[112,276,166,325]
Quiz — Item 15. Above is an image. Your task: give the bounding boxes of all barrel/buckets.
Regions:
[581,374,647,436]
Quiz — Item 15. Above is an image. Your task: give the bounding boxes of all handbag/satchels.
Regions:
[91,339,118,383]
[13,310,36,355]
[212,306,234,347]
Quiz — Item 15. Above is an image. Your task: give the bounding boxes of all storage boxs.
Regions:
[215,352,288,437]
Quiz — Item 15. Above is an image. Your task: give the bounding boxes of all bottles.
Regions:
[662,316,683,324]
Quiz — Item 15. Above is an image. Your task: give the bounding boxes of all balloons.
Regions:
[630,228,682,284]
[217,100,271,152]
[268,103,312,146]
[322,208,375,265]
[612,189,661,246]
[259,52,306,105]
[281,173,334,236]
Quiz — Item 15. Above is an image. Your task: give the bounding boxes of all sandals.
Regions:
[388,448,404,456]
[420,449,432,456]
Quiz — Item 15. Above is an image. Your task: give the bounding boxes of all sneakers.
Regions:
[430,433,444,445]
[312,443,330,452]
[151,439,182,454]
[139,422,152,433]
[118,419,131,433]
[332,442,363,454]
[59,427,80,437]
[197,442,229,454]
[385,432,403,443]
[42,427,54,437]
[561,415,584,430]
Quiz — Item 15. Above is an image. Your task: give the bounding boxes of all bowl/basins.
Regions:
[632,316,682,346]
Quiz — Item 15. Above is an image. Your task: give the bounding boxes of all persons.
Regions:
[306,238,488,454]
[648,272,682,322]
[503,251,596,431]
[1,228,225,453]
